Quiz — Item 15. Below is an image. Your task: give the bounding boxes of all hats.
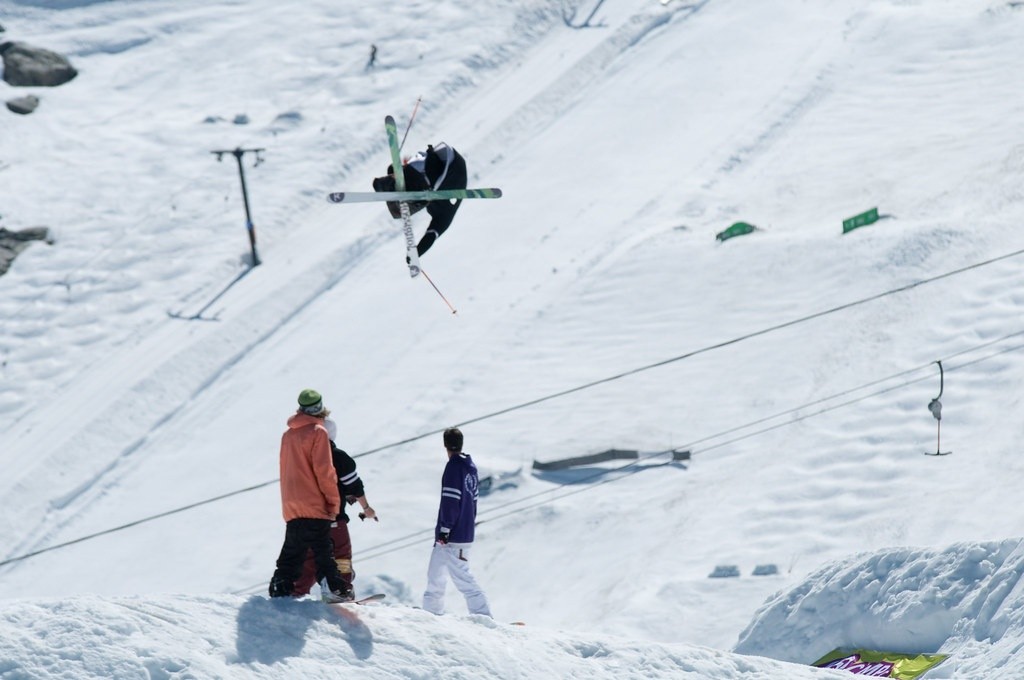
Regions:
[297,389,324,415]
[324,420,336,441]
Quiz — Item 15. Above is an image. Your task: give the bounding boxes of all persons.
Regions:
[268,390,354,601]
[387,142,468,258]
[294,418,376,595]
[423,427,492,618]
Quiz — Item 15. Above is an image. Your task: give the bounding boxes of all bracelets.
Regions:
[365,506,369,511]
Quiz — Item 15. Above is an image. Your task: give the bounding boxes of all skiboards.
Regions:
[317,576,387,610]
[320,113,502,280]
